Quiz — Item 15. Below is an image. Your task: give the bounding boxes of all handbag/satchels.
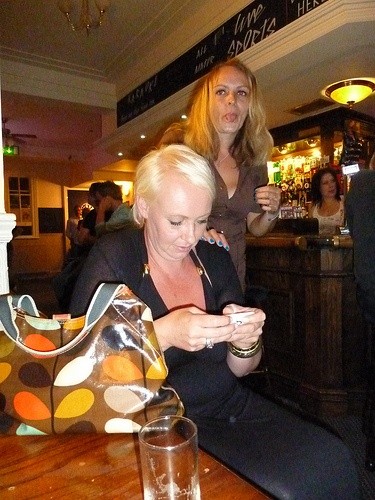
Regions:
[0,283,169,435]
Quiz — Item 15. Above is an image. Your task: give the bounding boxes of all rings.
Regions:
[207,337,214,349]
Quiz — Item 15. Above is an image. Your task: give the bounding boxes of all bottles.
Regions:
[273,154,321,207]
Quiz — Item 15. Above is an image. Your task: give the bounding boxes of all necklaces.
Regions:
[218,152,228,167]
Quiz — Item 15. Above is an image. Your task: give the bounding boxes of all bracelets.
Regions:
[265,211,279,221]
[227,338,262,358]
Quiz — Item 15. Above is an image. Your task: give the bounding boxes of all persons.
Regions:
[157,59,282,297]
[68,144,361,500]
[344,153,375,436]
[51,181,134,313]
[308,168,348,379]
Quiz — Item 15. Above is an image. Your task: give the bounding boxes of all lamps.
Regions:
[325,78,374,110]
[57,0,112,34]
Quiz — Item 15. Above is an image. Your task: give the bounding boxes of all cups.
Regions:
[138,414,201,500]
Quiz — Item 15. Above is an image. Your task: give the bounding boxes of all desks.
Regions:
[0,431,272,500]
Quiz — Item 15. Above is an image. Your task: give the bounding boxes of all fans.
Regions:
[2,117,37,145]
